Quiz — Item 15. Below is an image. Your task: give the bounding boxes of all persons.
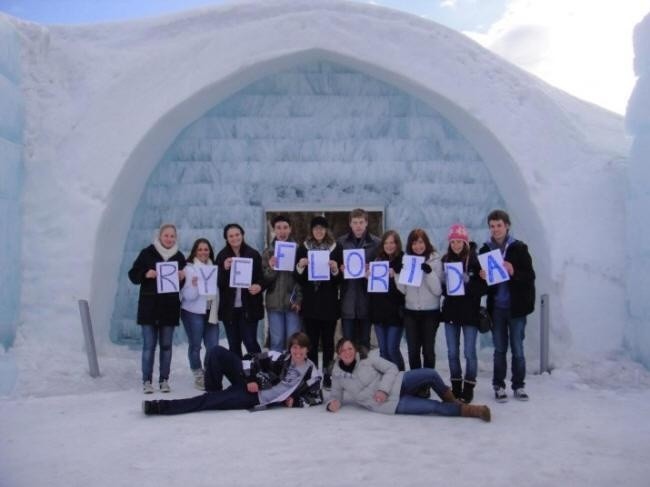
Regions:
[335,208,383,352]
[260,216,300,354]
[292,216,340,391]
[130,224,188,396]
[393,229,444,398]
[479,210,536,403]
[180,238,219,390]
[325,338,491,422]
[365,230,406,371]
[440,223,480,403]
[132,332,324,413]
[215,223,267,371]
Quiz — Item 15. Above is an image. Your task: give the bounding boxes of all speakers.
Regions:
[443,390,465,404]
[460,403,490,421]
[460,381,476,403]
[449,377,464,399]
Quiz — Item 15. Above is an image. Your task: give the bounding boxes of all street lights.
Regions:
[478,307,494,333]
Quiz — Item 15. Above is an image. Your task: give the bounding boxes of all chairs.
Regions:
[142,381,154,394]
[159,379,170,392]
[143,400,153,415]
[192,368,206,390]
[513,387,529,401]
[495,387,508,403]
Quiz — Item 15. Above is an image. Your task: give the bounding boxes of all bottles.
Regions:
[311,216,329,228]
[447,224,469,245]
